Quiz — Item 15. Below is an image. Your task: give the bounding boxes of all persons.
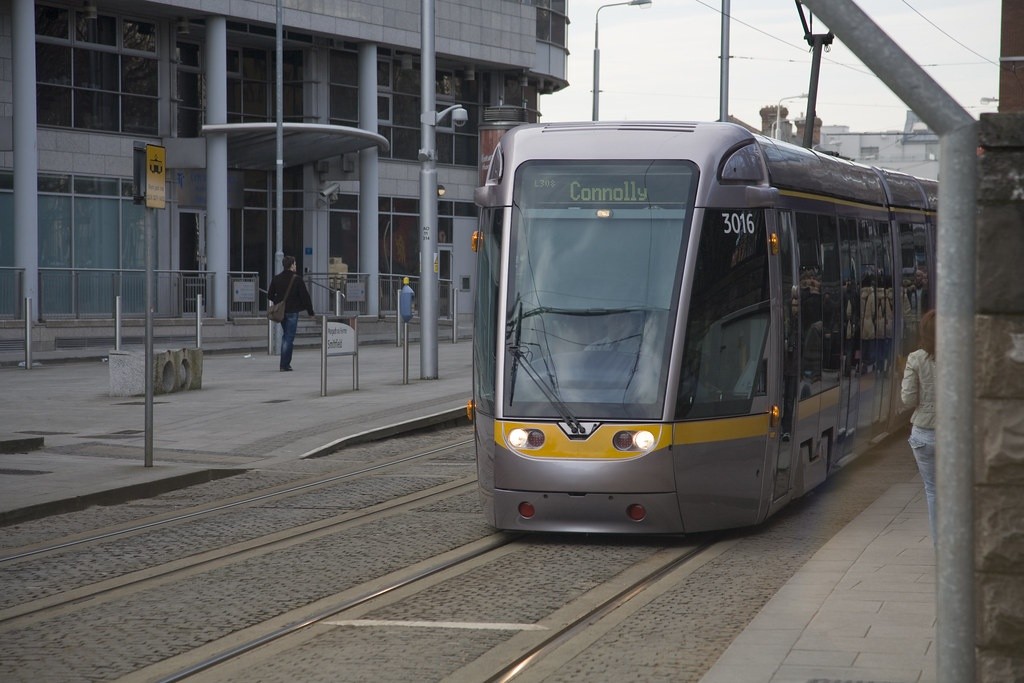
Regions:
[836,271,928,382]
[267,255,315,372]
[584,308,661,389]
[901,309,938,555]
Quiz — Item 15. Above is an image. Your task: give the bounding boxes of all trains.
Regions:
[465,117,936,546]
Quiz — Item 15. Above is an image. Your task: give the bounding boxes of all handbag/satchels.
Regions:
[267,301,287,322]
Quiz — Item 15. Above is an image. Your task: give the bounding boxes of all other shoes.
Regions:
[280,365,293,372]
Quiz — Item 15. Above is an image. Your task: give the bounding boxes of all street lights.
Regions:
[591,0,654,122]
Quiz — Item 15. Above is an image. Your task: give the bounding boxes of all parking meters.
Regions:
[399,276,416,384]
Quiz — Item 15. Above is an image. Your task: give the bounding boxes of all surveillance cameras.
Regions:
[451,109,469,127]
[319,183,340,197]
